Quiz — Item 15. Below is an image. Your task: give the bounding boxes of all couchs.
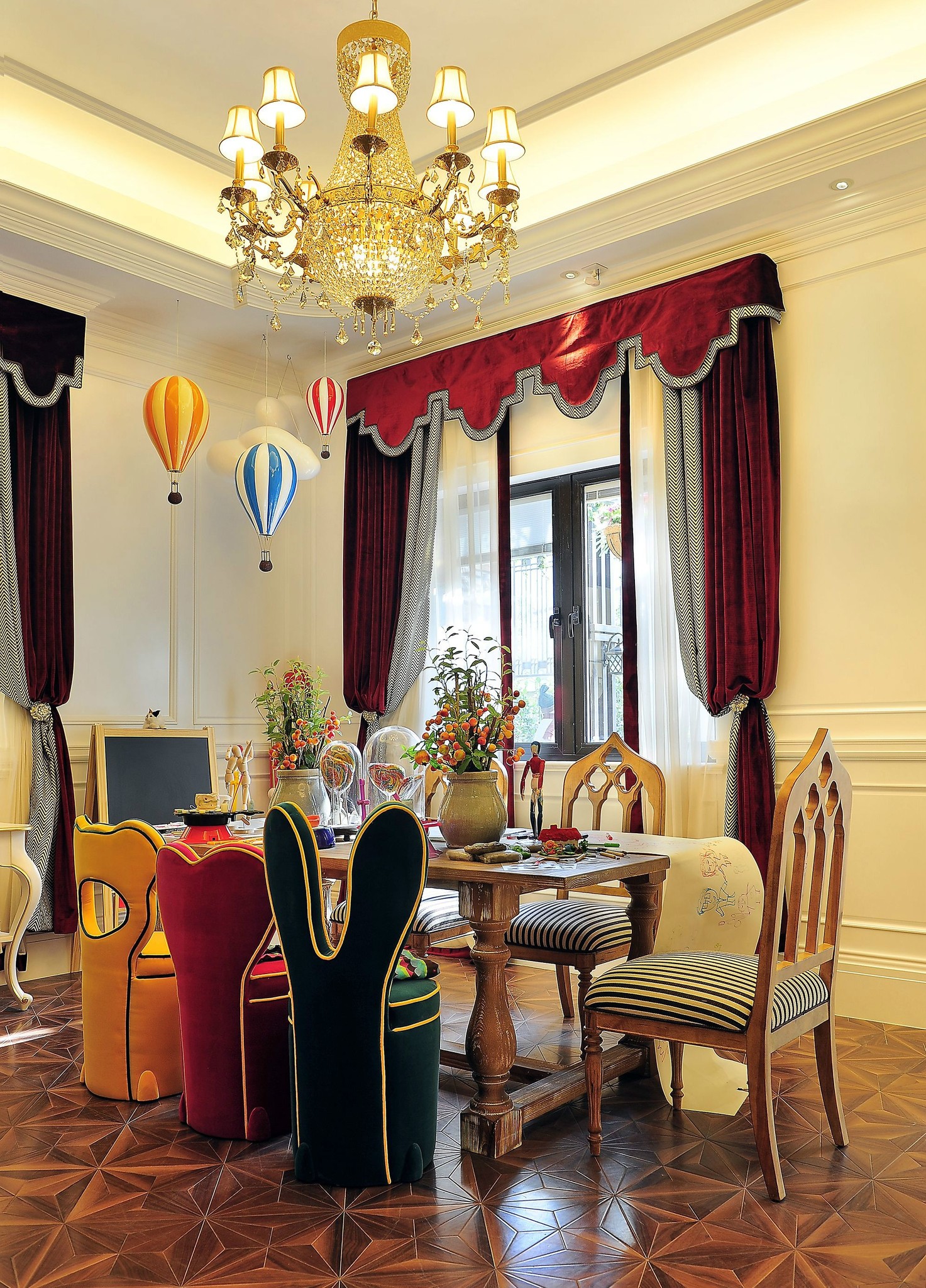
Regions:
[153,842,292,1143]
[262,800,442,1189]
[72,813,181,1102]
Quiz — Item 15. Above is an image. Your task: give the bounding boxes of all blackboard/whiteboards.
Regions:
[91,724,221,827]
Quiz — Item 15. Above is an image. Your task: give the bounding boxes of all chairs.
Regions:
[504,732,663,1063]
[579,725,851,1206]
[328,756,510,960]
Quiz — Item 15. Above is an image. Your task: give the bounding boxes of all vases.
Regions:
[437,771,510,848]
[268,769,331,826]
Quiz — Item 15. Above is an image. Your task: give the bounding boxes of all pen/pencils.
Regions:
[606,832,614,841]
[502,829,535,840]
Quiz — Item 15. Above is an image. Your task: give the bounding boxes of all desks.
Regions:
[149,816,672,1160]
[0,821,37,1012]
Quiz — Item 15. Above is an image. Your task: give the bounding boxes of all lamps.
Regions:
[213,1,525,359]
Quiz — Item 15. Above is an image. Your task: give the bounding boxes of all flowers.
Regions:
[399,620,526,773]
[252,658,358,770]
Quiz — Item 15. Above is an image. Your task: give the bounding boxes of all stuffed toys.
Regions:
[142,708,167,730]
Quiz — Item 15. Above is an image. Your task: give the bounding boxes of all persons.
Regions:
[231,739,254,810]
[519,741,546,839]
[218,744,244,811]
